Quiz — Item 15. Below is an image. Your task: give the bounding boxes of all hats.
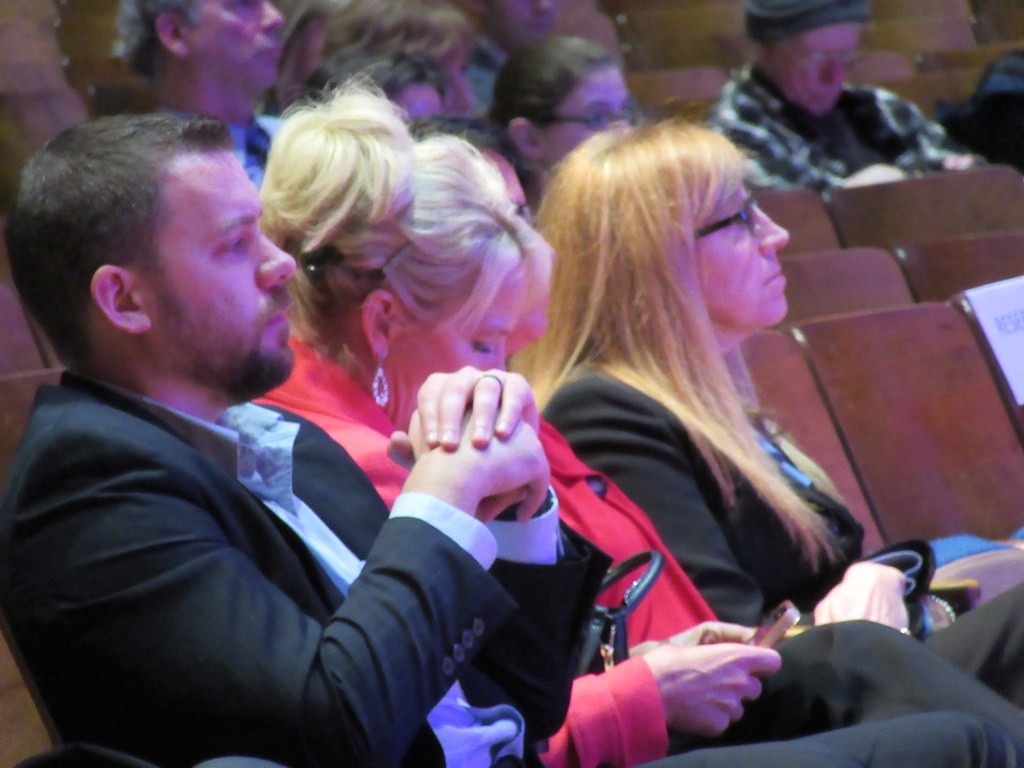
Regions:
[744,0,870,42]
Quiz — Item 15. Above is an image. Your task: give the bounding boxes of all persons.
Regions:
[107,1,1023,225]
[505,118,1024,705]
[253,75,1024,767]
[1,114,1022,768]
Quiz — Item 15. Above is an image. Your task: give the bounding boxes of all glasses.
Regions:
[693,192,762,240]
[528,108,633,130]
[811,50,871,73]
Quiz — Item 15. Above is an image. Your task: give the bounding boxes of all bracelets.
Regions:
[932,594,957,623]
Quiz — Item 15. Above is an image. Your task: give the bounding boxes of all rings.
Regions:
[900,627,911,636]
[477,375,505,394]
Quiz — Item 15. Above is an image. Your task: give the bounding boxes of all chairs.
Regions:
[0,0,1024,768]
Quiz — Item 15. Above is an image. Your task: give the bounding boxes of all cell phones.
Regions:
[746,599,801,648]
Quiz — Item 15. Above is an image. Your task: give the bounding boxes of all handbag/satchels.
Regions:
[577,549,663,679]
